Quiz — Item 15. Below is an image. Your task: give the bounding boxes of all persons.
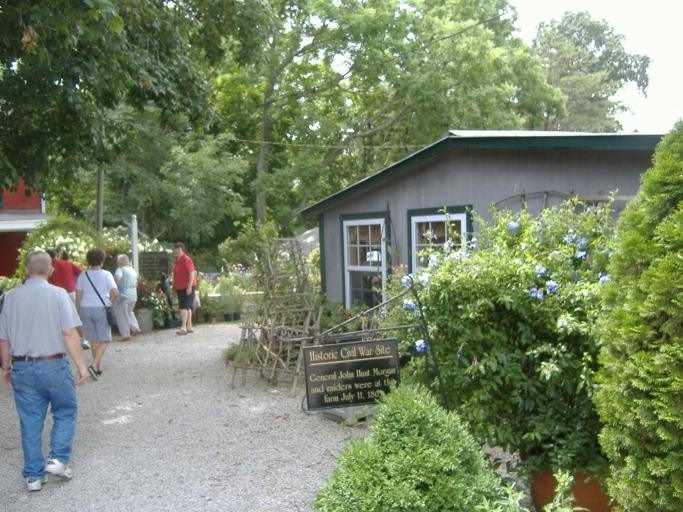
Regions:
[0,250,91,493]
[57,251,69,261]
[113,254,142,344]
[41,248,90,349]
[73,247,121,383]
[170,241,197,336]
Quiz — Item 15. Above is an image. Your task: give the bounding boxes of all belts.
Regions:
[13,353,64,363]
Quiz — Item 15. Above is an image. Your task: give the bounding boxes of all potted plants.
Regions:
[135,300,154,334]
[203,301,244,325]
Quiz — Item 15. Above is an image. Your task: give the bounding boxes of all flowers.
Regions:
[385,183,616,453]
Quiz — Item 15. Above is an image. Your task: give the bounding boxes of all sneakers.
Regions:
[88,365,104,381]
[82,340,91,349]
[176,328,194,334]
[26,473,49,492]
[44,457,73,479]
[117,328,142,342]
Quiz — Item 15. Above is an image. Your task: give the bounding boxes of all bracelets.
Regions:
[1,366,11,372]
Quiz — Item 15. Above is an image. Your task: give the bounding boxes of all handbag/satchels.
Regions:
[105,306,117,325]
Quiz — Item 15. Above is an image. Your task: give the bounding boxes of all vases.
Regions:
[526,451,617,510]
